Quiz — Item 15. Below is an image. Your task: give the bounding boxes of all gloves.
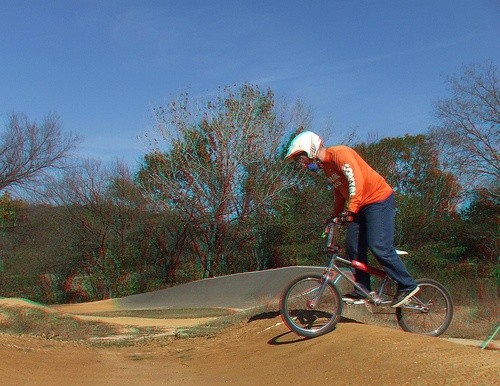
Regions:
[323,216,336,229]
[339,211,355,225]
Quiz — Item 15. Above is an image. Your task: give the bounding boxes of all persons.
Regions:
[285,130,421,307]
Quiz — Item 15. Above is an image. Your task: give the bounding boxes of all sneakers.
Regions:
[342,290,376,303]
[390,285,420,308]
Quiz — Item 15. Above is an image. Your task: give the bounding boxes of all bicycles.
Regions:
[278,212,453,338]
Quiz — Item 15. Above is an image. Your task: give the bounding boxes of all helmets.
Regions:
[284,131,322,159]
